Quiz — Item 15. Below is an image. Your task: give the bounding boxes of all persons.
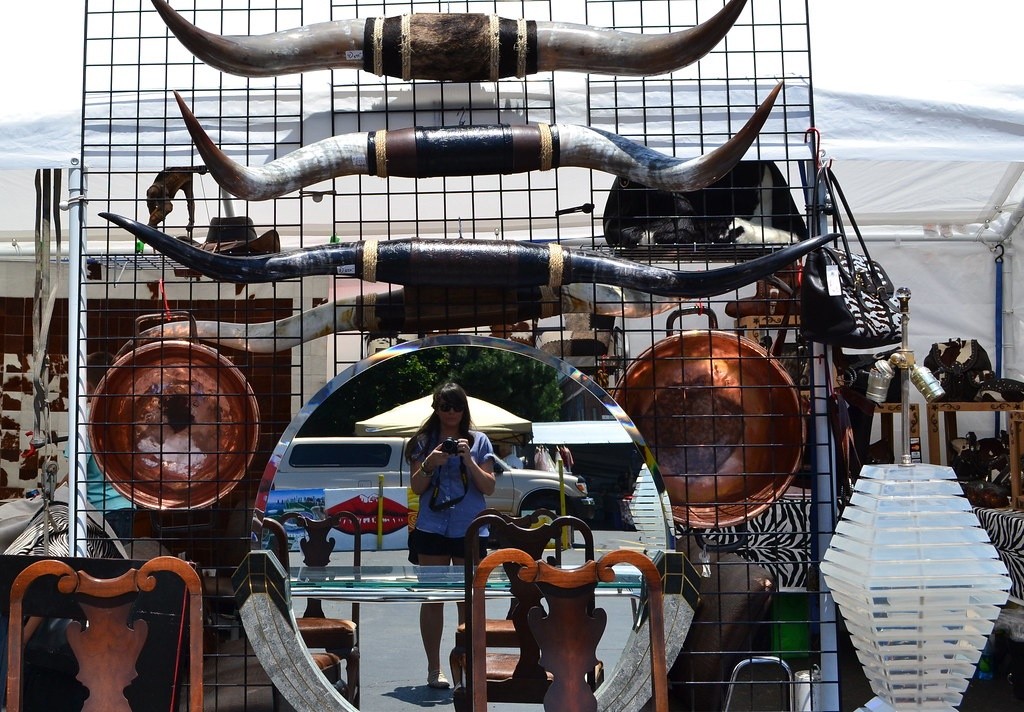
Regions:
[403,382,496,688]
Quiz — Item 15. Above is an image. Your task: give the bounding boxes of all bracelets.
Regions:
[420,461,436,473]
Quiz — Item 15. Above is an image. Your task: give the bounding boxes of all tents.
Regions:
[353,388,533,462]
[1,0,1023,712]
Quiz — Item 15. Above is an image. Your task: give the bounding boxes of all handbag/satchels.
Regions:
[841,478,855,506]
[946,429,1024,490]
[923,337,994,401]
[799,167,906,346]
[840,348,900,403]
[408,529,418,565]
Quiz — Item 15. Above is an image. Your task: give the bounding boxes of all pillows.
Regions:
[3,481,128,559]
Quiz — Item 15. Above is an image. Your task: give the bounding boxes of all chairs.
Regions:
[639,535,779,712]
[5,555,204,712]
[262,516,350,712]
[450,508,562,686]
[471,548,668,712]
[453,515,596,712]
[273,512,360,711]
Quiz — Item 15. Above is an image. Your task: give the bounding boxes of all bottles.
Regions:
[910,361,945,403]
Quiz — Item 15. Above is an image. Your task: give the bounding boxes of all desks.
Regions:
[290,566,642,623]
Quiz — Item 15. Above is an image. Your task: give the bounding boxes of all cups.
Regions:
[866,368,890,403]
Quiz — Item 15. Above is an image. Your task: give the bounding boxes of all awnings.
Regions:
[530,418,634,445]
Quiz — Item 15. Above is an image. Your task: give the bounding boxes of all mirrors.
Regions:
[231,334,703,712]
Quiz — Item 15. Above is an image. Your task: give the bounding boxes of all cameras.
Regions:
[441,436,464,456]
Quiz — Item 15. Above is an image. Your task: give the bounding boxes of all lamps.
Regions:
[819,287,1013,712]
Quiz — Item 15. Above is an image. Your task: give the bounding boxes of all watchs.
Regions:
[419,467,434,478]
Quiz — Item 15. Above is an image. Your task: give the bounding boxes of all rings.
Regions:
[462,446,465,450]
[439,454,443,457]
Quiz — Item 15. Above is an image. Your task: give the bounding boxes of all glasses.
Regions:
[439,403,463,412]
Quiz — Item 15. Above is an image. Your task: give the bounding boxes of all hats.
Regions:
[175,216,282,253]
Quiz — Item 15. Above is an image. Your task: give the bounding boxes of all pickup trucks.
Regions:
[272,435,596,544]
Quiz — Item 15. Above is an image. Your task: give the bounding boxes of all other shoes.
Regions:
[428,670,450,687]
